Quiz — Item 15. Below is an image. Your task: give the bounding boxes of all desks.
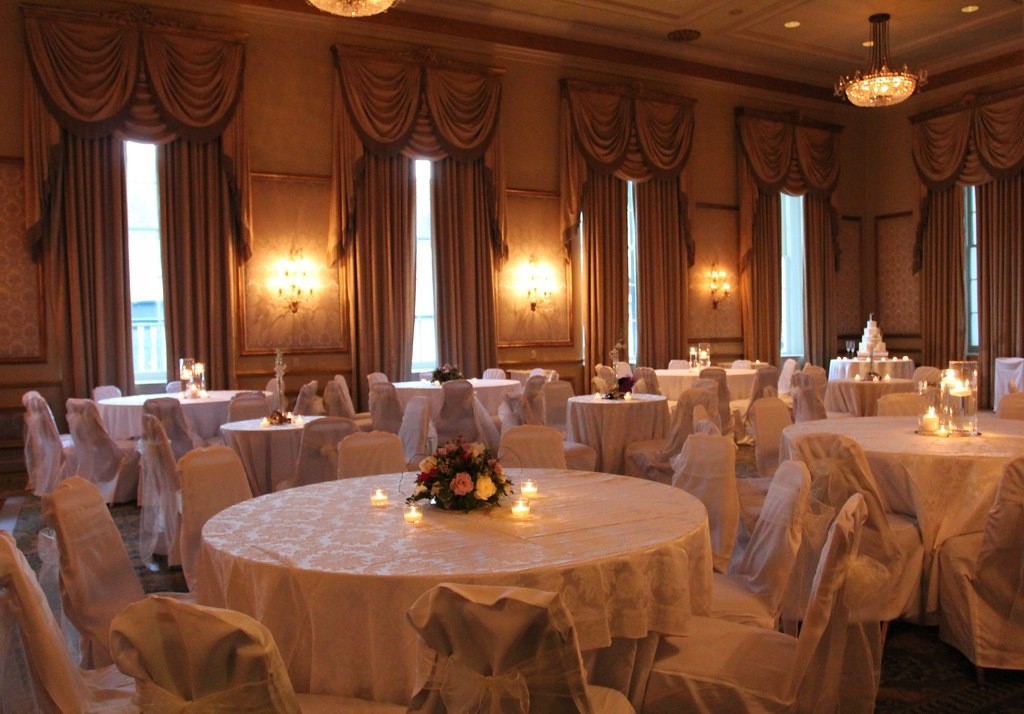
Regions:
[993,358,1024,412]
[778,417,1024,626]
[655,369,757,401]
[98,391,275,440]
[824,379,919,417]
[198,468,713,714]
[567,394,670,476]
[220,416,327,497]
[828,359,915,382]
[392,379,522,422]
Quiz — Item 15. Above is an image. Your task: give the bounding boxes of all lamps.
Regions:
[833,12,928,107]
[306,0,405,18]
[707,261,730,310]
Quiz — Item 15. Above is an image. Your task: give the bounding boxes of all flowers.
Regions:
[431,364,467,384]
[605,376,639,400]
[405,433,514,516]
[267,408,292,425]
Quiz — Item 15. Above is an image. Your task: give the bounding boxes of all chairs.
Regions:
[0,360,1024,714]
